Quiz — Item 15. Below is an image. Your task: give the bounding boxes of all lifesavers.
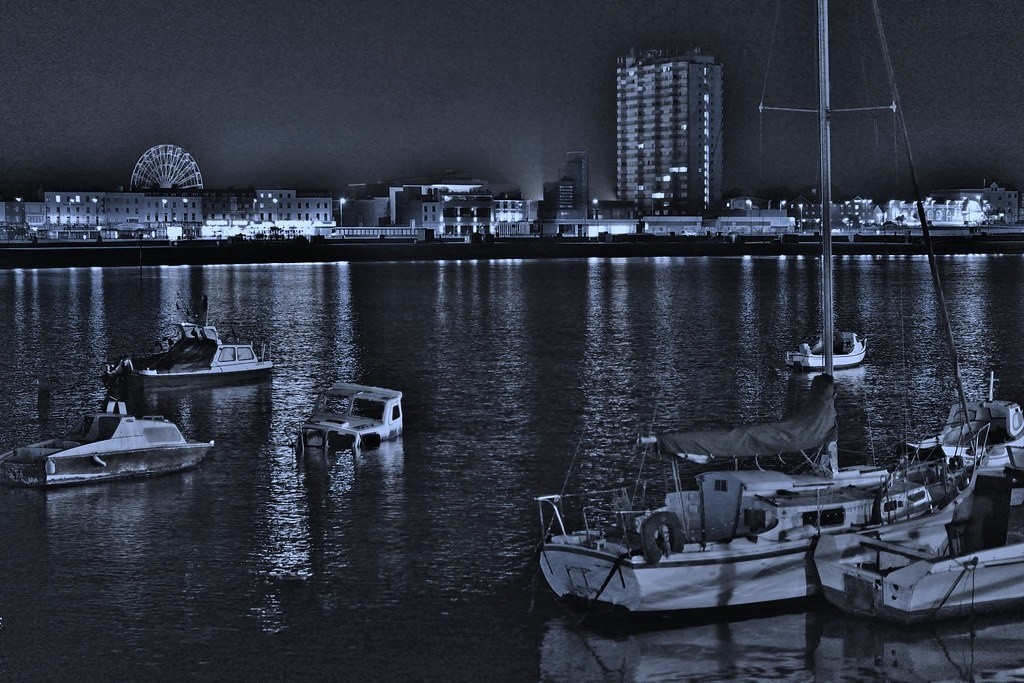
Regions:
[640,510,684,566]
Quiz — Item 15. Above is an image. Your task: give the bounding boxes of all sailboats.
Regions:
[532,0,990,626]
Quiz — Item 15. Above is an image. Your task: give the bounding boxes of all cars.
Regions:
[681,230,697,236]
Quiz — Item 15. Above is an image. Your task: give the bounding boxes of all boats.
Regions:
[102,322,273,397]
[0,400,215,486]
[812,445,1024,630]
[785,331,867,371]
[299,382,404,449]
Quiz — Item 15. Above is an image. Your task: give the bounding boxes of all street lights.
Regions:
[273,199,278,221]
[183,198,189,230]
[340,199,345,235]
[162,199,167,238]
[799,204,803,230]
[747,200,752,233]
[780,200,787,237]
[16,198,24,240]
